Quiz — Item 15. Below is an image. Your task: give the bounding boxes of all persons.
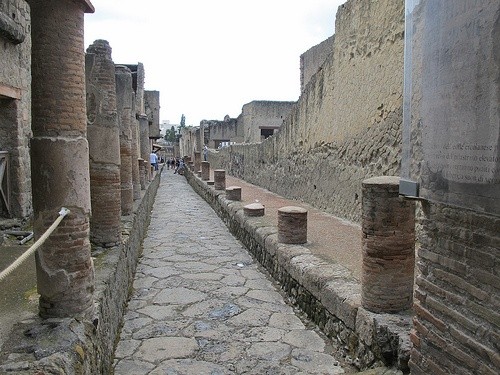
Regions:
[165,156,185,174]
[202,143,207,161]
[150,150,158,171]
[217,141,223,148]
